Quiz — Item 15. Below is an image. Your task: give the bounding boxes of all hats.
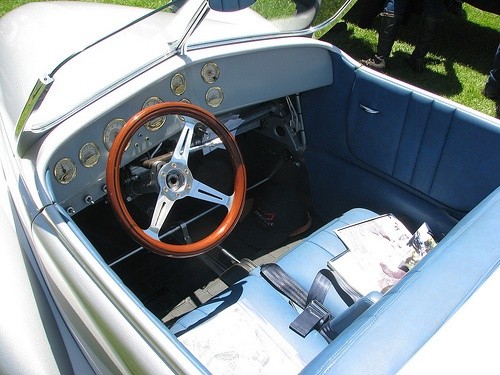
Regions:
[240,182,307,250]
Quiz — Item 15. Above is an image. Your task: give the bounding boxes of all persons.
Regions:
[359,0,443,71]
[482,43,500,120]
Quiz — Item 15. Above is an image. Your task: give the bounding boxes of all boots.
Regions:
[409,12,442,75]
[363,11,401,68]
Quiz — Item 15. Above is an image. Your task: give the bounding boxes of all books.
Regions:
[327,213,439,296]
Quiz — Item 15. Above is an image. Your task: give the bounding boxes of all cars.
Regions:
[0,0,500,375]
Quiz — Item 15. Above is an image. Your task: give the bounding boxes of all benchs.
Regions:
[168,182,500,375]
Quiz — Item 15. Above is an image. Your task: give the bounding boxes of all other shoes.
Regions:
[483,83,500,116]
